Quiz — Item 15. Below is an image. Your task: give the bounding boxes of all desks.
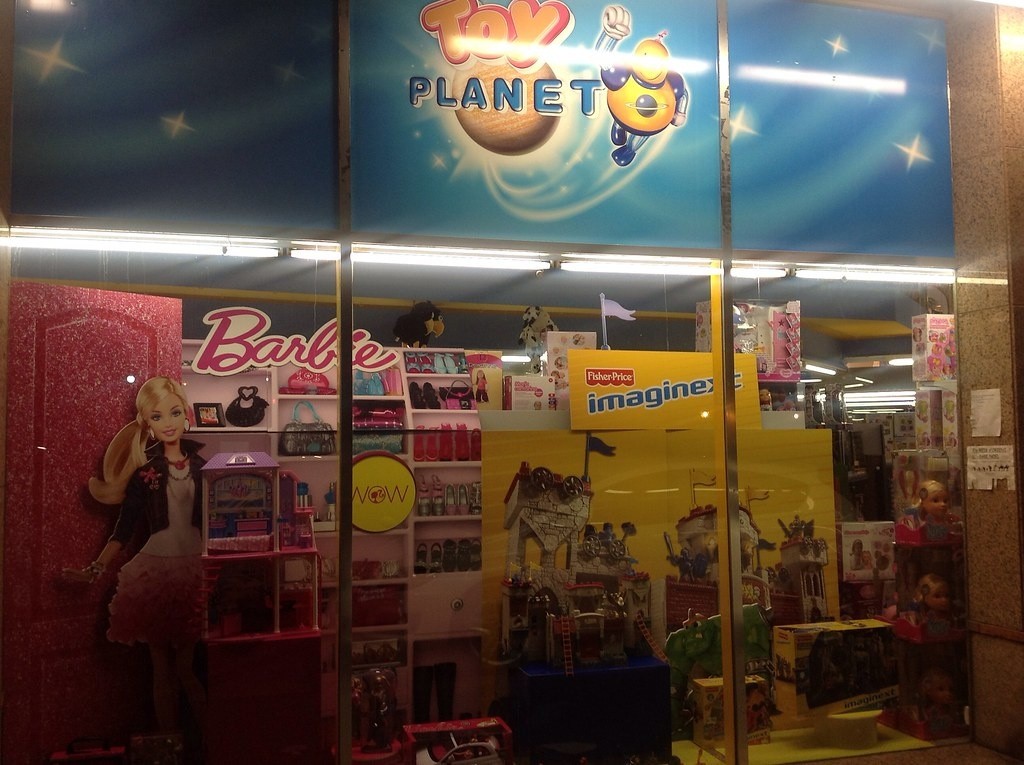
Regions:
[514,647,672,757]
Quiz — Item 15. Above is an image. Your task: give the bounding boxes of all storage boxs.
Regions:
[834,520,897,583]
[540,331,597,410]
[464,350,503,410]
[693,674,770,747]
[693,299,801,381]
[771,618,902,722]
[505,375,556,411]
[878,313,972,743]
[759,381,808,429]
[402,717,515,765]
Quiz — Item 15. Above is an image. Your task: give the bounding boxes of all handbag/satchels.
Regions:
[441,380,476,409]
[352,433,404,454]
[352,558,381,579]
[282,401,334,453]
[354,371,385,395]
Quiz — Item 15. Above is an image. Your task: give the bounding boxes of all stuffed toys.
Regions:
[393,301,434,350]
[516,306,559,374]
[409,308,444,348]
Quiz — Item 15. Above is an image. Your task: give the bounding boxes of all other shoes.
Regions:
[413,543,428,573]
[434,353,447,373]
[445,484,457,515]
[458,483,469,515]
[417,353,433,372]
[457,539,470,570]
[410,382,426,409]
[430,543,442,573]
[471,539,480,569]
[406,352,419,372]
[445,355,457,374]
[424,382,441,409]
[444,539,456,572]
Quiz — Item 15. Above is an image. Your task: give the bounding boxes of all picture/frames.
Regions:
[193,402,226,427]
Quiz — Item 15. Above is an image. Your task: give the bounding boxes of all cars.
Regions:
[409,456,868,681]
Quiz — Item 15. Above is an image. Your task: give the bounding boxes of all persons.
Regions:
[900,477,966,729]
[759,388,771,411]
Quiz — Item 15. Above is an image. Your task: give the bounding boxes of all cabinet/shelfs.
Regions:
[182,365,487,765]
